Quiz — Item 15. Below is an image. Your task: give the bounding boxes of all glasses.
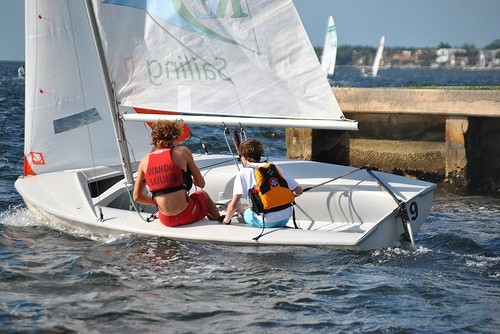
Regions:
[239,154,243,158]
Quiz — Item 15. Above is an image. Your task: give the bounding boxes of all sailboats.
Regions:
[318,14,386,85]
[13,1,437,252]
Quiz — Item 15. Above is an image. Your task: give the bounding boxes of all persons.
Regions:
[132,119,221,227]
[217,139,304,228]
[17,64,25,76]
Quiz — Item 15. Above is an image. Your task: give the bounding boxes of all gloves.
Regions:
[218,215,231,224]
